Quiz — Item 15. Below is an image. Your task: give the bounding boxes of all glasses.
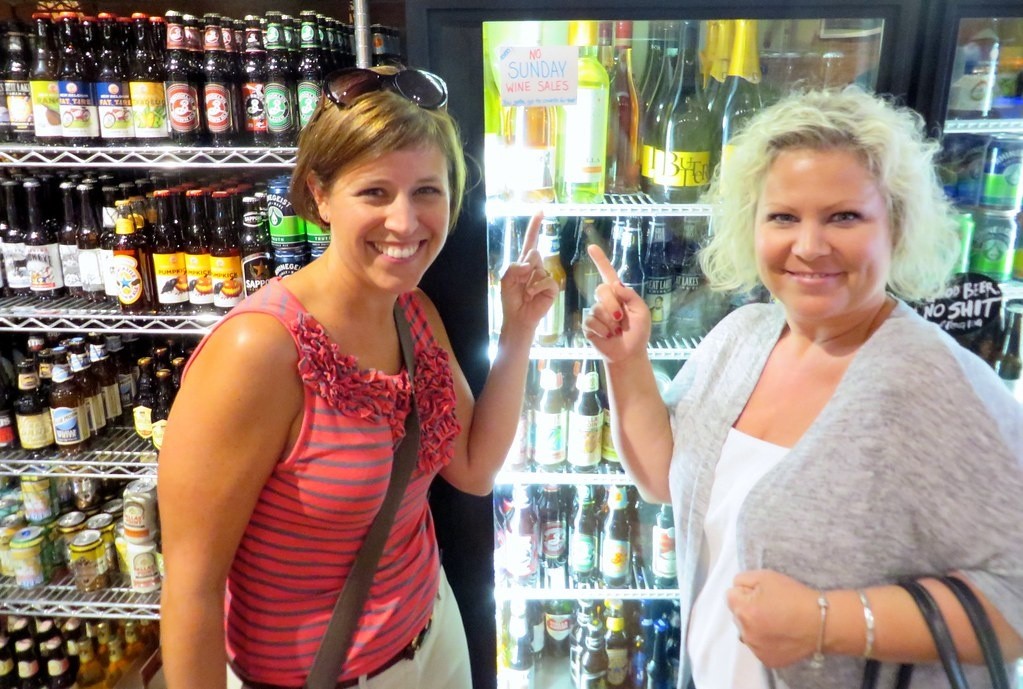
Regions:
[311,69,448,128]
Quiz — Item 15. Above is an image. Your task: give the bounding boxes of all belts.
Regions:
[335,617,432,689]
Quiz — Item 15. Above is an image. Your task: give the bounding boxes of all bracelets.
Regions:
[857,589,874,658]
[808,589,828,668]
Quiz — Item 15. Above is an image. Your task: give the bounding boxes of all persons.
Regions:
[157,63,560,689]
[582,85,1023,689]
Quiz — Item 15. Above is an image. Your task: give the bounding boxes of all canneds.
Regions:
[937,134,1023,281]
[265,173,330,277]
[0,471,164,593]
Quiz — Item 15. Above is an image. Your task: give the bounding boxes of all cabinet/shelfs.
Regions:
[0,0,372,620]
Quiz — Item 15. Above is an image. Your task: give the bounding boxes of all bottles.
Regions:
[1,610,160,689]
[0,152,276,311]
[0,314,206,465]
[481,18,846,689]
[957,298,1023,395]
[0,8,403,147]
